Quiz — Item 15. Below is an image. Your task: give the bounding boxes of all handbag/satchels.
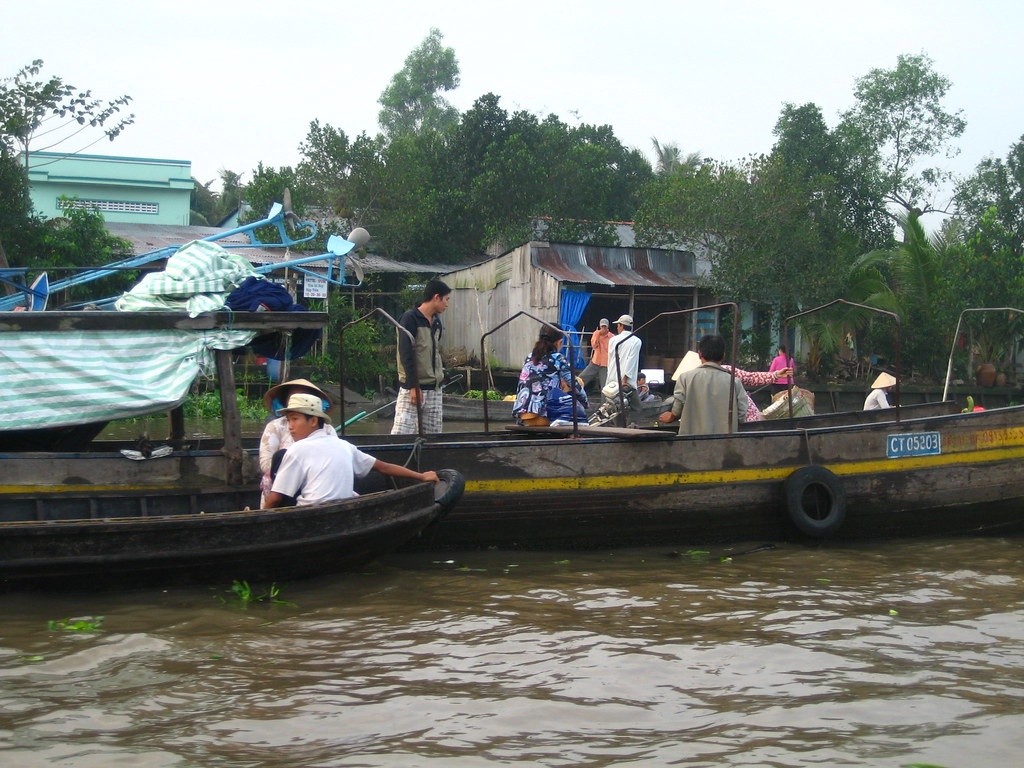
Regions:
[545,385,589,423]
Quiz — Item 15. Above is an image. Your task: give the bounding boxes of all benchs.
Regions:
[504,425,678,439]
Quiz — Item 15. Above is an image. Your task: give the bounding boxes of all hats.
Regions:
[612,314,633,326]
[599,318,609,326]
[276,393,332,424]
[871,372,896,388]
[263,379,332,408]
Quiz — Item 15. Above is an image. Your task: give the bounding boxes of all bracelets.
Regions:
[773,370,780,379]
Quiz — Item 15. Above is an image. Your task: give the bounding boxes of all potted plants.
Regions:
[932,205,1024,387]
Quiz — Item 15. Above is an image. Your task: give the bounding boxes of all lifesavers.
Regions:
[773,466,850,544]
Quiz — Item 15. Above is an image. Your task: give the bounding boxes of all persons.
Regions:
[637,373,655,403]
[512,322,590,439]
[259,379,339,510]
[863,371,902,411]
[671,351,793,423]
[578,318,616,408]
[659,334,749,435]
[605,315,643,428]
[264,393,440,510]
[769,344,796,406]
[390,279,451,435]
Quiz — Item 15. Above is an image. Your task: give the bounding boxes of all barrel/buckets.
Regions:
[673,358,682,371]
[661,358,673,374]
[642,353,663,369]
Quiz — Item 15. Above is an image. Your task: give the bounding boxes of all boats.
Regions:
[0,454,466,595]
[1,296,1024,547]
